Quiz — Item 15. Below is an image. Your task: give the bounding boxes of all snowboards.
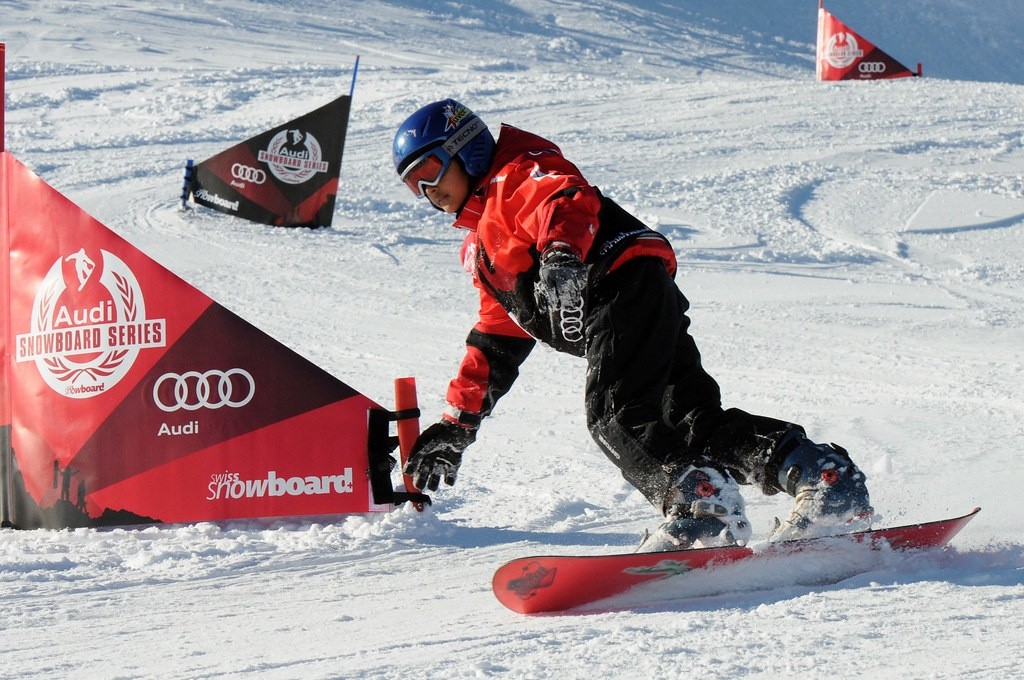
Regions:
[491,503,985,617]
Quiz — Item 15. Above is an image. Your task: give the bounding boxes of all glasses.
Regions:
[401,148,453,198]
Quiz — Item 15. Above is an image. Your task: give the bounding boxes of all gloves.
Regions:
[401,419,481,492]
[533,247,589,351]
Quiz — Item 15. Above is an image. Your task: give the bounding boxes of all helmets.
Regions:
[393,98,495,178]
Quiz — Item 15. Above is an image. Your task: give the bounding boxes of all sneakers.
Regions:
[769,438,874,542]
[634,468,752,553]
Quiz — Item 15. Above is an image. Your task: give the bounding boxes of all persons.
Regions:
[392,98,871,557]
